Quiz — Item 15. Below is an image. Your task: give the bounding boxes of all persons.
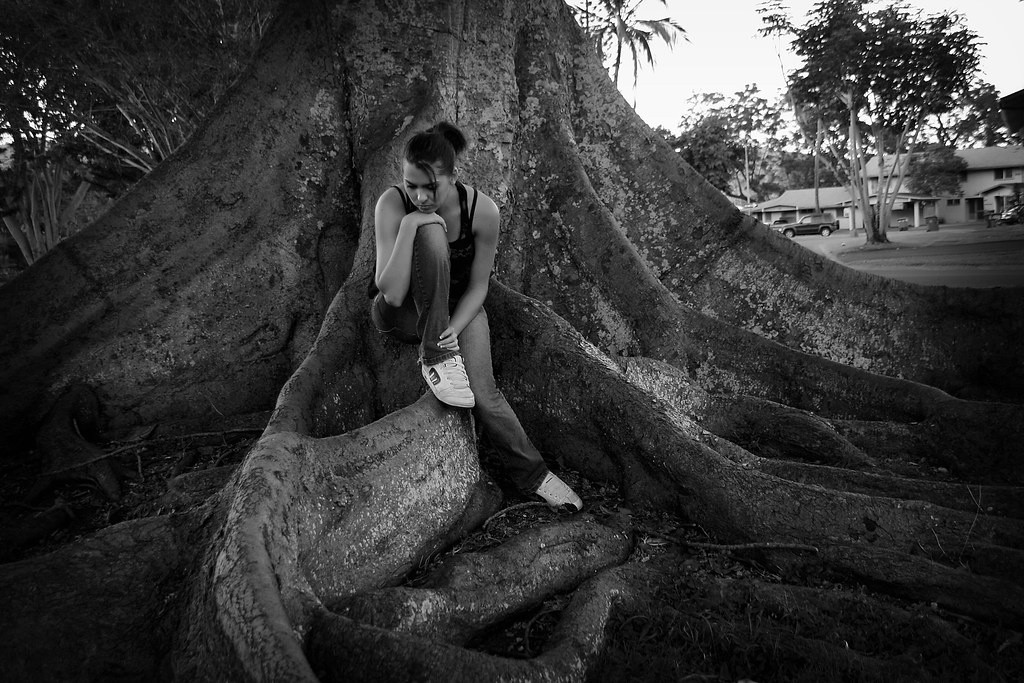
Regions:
[372,122,582,516]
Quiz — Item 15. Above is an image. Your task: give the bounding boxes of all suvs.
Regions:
[783,212,840,238]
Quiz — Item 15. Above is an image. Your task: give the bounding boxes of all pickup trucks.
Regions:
[770,219,788,234]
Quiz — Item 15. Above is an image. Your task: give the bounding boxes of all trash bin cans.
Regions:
[925,216,938,230]
[896,218,907,230]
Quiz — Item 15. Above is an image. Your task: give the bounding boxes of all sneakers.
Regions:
[525,469,584,515]
[421,355,475,408]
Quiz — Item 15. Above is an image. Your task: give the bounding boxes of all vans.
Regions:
[1000,203,1024,225]
